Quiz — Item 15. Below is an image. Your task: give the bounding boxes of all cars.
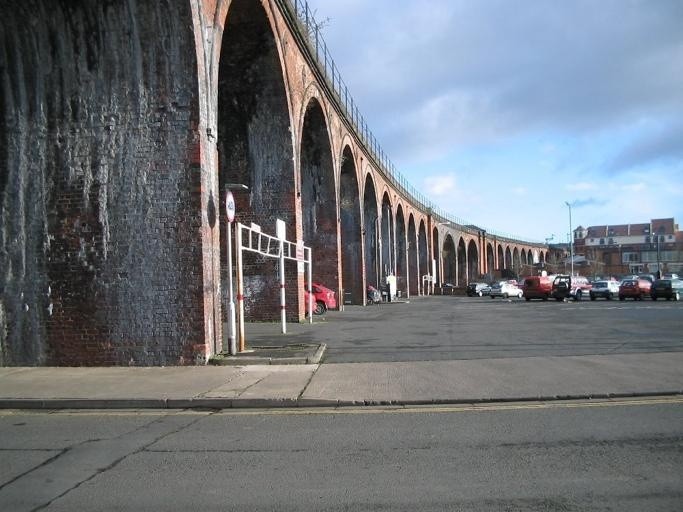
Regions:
[301,282,337,315]
[442,282,455,287]
[463,269,683,303]
[365,283,382,306]
[301,290,317,318]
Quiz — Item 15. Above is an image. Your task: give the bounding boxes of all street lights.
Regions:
[650,230,662,281]
[437,221,451,292]
[564,200,575,277]
